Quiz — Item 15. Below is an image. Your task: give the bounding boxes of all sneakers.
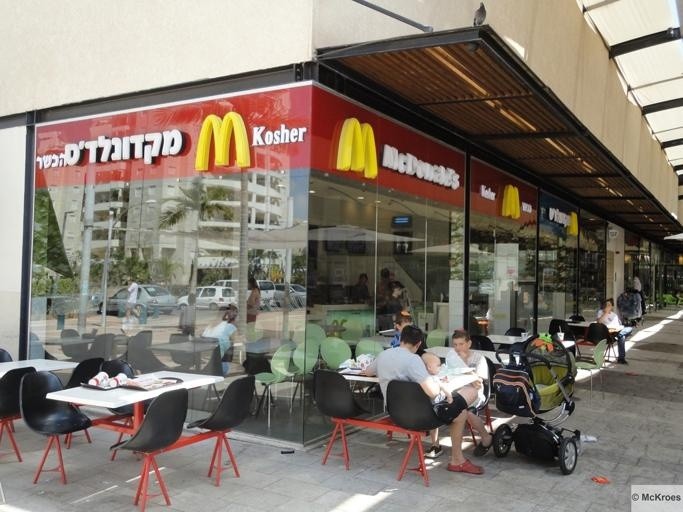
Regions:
[425,445,443,458]
[474,433,494,456]
[617,359,628,364]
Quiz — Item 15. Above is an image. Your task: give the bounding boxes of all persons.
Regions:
[119,267,264,378]
[348,273,370,302]
[598,298,634,365]
[388,316,422,353]
[364,325,485,475]
[421,352,453,407]
[634,270,647,315]
[375,267,396,332]
[466,299,488,335]
[424,330,497,458]
[380,281,411,332]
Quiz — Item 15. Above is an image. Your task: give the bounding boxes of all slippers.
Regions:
[447,459,484,474]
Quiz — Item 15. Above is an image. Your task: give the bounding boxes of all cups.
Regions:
[557,332,564,342]
[88,370,127,388]
[521,333,527,340]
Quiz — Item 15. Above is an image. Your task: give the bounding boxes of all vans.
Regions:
[212,277,275,308]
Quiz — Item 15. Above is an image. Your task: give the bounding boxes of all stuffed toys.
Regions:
[531,332,555,353]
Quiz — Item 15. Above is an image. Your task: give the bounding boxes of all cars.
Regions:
[100,284,175,315]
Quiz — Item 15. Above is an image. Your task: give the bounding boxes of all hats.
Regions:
[392,311,411,322]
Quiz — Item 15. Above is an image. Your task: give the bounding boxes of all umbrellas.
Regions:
[177,220,425,255]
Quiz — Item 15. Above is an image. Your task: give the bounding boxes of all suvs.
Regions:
[179,286,232,309]
[277,281,306,305]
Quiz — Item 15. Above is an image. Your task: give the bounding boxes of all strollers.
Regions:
[617,290,643,327]
[494,353,581,475]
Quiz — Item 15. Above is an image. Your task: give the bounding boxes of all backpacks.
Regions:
[493,346,541,417]
